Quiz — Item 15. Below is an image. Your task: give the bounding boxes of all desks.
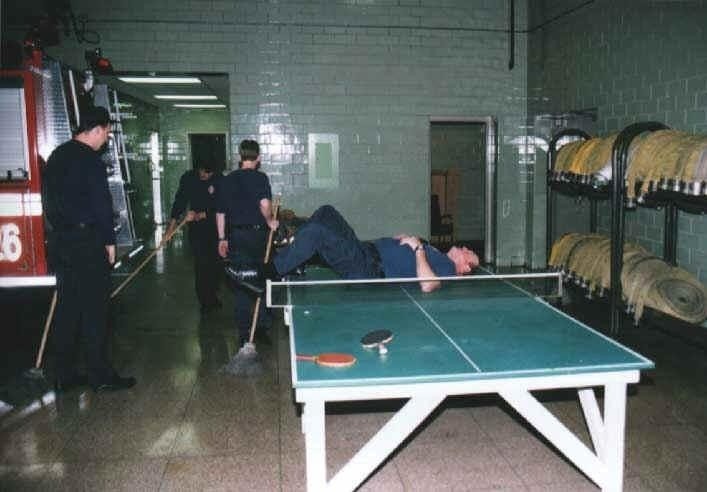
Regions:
[265,253,654,492]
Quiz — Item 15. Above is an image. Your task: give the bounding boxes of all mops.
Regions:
[218,192,282,376]
[0,288,58,408]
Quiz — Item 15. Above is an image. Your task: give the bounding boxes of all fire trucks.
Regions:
[0,23,142,290]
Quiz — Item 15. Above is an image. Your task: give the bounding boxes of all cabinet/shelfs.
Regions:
[546,120,705,349]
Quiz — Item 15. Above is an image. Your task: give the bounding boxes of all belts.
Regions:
[368,241,385,279]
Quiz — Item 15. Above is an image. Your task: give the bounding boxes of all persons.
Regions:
[163,142,228,316]
[39,105,139,392]
[214,139,280,346]
[222,203,479,292]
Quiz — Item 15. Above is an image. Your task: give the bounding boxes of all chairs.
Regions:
[431,195,455,249]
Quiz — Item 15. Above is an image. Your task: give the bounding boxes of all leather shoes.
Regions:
[223,261,266,294]
[91,377,137,392]
[56,374,88,391]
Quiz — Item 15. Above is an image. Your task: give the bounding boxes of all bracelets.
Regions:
[217,238,227,241]
[413,242,423,252]
[206,207,209,224]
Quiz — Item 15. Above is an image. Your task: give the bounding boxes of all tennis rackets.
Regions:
[298,354,356,367]
[361,329,394,354]
